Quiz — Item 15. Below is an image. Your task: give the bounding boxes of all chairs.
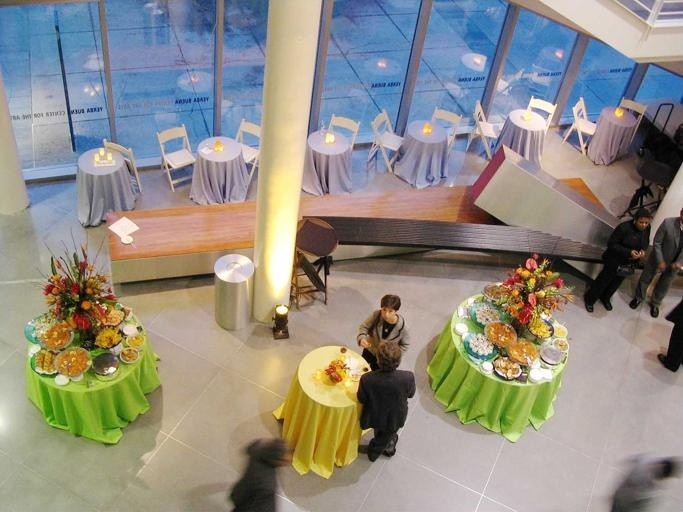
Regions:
[431,107,463,160]
[153,103,181,132]
[157,124,196,193]
[618,97,648,144]
[466,100,500,160]
[235,119,262,182]
[328,113,360,153]
[290,218,338,310]
[103,139,142,193]
[435,80,466,112]
[335,88,371,121]
[367,110,404,174]
[527,96,558,133]
[526,64,556,100]
[562,97,597,156]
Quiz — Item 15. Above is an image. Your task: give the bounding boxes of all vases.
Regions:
[512,318,526,337]
[78,331,95,348]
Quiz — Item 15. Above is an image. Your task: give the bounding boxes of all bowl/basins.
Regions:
[527,369,542,382]
[54,375,69,385]
[481,362,493,371]
[455,324,468,334]
[123,324,136,335]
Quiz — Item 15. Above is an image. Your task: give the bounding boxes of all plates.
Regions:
[464,336,496,360]
[30,348,63,377]
[526,318,554,339]
[92,352,118,375]
[348,369,362,381]
[552,338,568,353]
[553,325,567,337]
[484,320,517,348]
[53,347,91,376]
[100,306,126,327]
[70,375,84,382]
[540,346,561,364]
[25,316,53,344]
[481,282,506,301]
[493,355,521,379]
[125,334,144,349]
[119,347,139,363]
[508,341,537,365]
[470,304,509,329]
[93,327,123,349]
[37,324,73,350]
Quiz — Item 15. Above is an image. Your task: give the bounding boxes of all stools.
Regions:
[619,160,675,220]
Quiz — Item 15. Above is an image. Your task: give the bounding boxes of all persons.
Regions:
[356,294,410,369]
[226,437,303,512]
[358,341,417,462]
[629,208,683,318]
[605,452,682,512]
[656,299,683,372]
[583,207,654,320]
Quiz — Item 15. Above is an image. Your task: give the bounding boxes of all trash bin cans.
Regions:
[213,254,254,331]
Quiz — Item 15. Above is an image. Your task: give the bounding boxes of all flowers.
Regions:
[33,232,116,333]
[497,257,575,328]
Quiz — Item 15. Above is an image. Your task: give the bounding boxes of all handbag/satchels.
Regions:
[616,260,636,277]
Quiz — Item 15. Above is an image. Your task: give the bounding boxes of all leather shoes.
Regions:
[603,301,613,311]
[657,353,671,371]
[585,303,594,313]
[630,298,641,309]
[650,305,659,317]
